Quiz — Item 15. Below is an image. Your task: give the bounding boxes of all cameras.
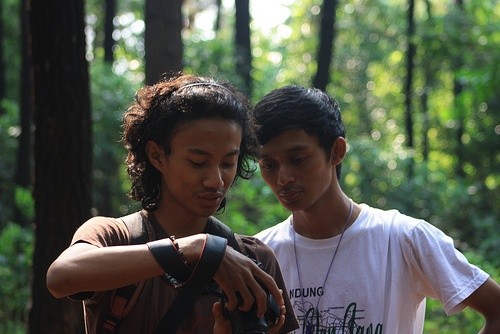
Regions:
[221,259,281,334]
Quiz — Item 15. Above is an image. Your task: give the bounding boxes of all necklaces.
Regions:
[289,196,355,334]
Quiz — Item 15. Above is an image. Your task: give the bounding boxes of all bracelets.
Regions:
[170,235,194,270]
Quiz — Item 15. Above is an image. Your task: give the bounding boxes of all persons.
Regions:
[247,84,500,334]
[45,74,300,334]
